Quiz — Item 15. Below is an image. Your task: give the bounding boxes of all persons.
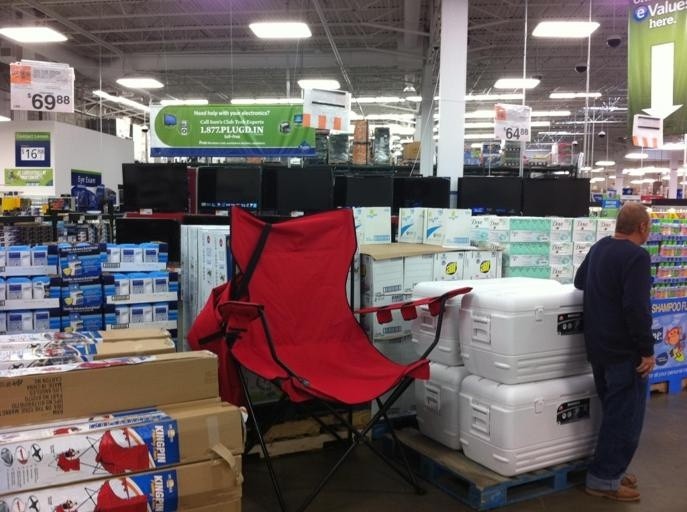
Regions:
[574,202,656,501]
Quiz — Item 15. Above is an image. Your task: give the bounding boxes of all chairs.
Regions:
[188,205,471,512]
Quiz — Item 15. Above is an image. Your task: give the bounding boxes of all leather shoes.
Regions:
[585,485,641,501]
[621,473,639,488]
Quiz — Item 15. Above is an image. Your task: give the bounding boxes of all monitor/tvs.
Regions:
[196,166,262,214]
[456,176,589,217]
[121,161,189,213]
[294,114,303,122]
[163,113,177,125]
[264,160,451,214]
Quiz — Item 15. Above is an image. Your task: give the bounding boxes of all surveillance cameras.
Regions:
[598,130,606,138]
[575,61,587,73]
[572,140,578,146]
[433,43,438,49]
[142,126,148,132]
[607,35,621,48]
[532,72,544,79]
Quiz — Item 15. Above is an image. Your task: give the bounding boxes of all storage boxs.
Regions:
[181,208,617,333]
[413,360,464,453]
[370,335,416,421]
[460,280,592,382]
[461,374,604,477]
[0,329,247,512]
[409,278,463,366]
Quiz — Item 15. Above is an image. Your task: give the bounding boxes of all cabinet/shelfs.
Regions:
[0,191,178,331]
